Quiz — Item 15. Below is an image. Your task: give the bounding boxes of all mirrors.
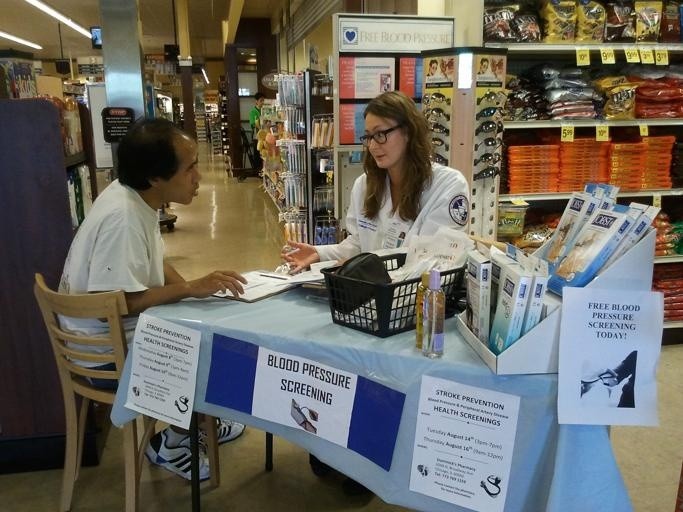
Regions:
[236,48,257,70]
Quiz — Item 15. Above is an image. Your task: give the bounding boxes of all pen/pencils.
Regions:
[260,274,287,280]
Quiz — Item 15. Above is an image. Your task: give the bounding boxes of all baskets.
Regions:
[320,254,467,338]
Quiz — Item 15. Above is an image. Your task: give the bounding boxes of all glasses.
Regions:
[360,125,401,146]
[423,93,450,163]
[474,91,506,181]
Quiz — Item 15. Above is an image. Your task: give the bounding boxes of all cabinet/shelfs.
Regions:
[86,82,114,172]
[0,98,97,462]
[259,69,334,245]
[445,0,683,329]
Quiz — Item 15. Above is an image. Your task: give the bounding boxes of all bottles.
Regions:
[415,267,445,359]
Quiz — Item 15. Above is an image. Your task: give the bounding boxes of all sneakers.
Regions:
[198,418,246,447]
[144,429,210,481]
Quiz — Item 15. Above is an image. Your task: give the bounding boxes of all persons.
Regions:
[427,60,438,76]
[279,90,470,497]
[249,92,266,178]
[477,58,489,74]
[603,352,636,408]
[55,116,248,483]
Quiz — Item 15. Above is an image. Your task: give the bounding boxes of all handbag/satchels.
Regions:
[332,253,392,314]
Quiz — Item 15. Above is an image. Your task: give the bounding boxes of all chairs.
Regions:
[33,273,219,512]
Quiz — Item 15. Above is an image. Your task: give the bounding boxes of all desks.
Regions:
[110,259,635,512]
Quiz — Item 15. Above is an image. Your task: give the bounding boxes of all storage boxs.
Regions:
[497,202,530,238]
